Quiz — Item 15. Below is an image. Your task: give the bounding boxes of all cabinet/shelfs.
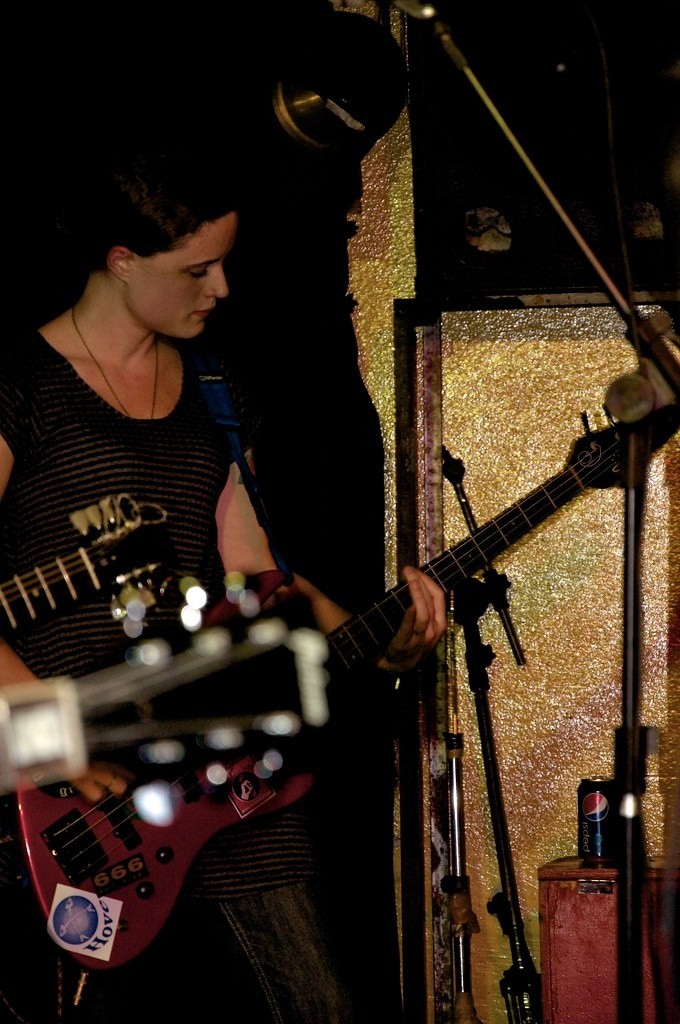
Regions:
[537,850,680,1024]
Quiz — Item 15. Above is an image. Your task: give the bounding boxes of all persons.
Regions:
[0,157,446,1024]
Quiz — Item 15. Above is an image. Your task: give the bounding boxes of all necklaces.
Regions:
[71,305,159,420]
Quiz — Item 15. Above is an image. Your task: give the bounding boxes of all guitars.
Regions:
[0,403,658,972]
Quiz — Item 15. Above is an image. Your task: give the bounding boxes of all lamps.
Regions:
[270,1,679,429]
